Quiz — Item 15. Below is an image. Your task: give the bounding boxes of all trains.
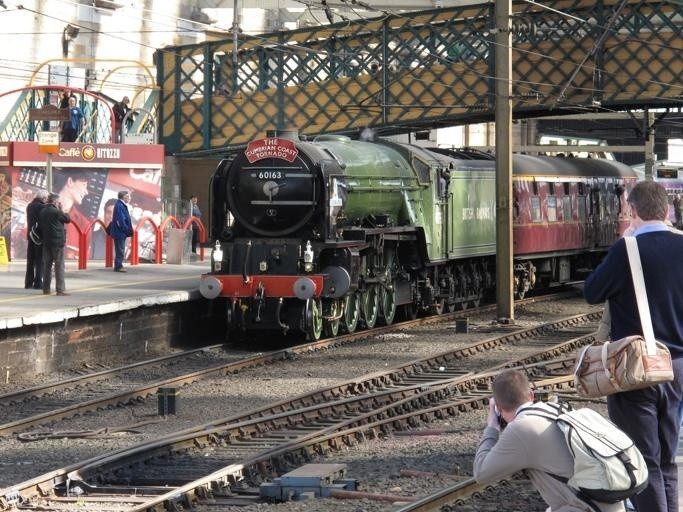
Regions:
[199,127,682,343]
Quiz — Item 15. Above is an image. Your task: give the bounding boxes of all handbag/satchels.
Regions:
[104,220,111,235]
[29,222,44,248]
[572,334,674,398]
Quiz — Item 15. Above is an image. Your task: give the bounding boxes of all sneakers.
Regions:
[24,285,70,296]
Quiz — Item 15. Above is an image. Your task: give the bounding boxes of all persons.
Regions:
[110,96,133,143]
[584,181,683,511]
[25,191,48,289]
[52,168,92,260]
[39,193,70,295]
[183,194,201,253]
[92,198,116,259]
[473,369,628,512]
[60,94,85,142]
[110,190,133,273]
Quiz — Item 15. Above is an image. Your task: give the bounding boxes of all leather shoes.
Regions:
[115,267,128,272]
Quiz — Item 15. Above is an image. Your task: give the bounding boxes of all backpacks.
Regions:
[511,401,652,505]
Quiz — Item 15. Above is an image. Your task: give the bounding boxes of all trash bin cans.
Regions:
[170,229,194,265]
